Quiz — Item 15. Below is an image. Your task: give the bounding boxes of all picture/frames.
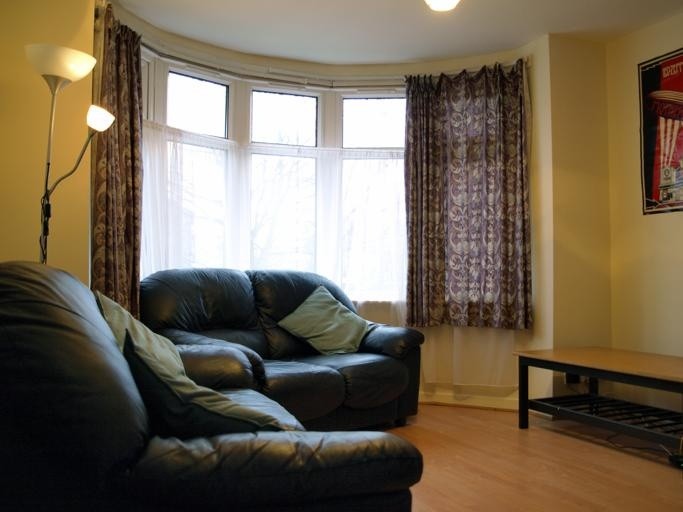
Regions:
[636,46,683,216]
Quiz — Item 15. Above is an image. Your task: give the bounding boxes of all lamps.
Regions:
[23,40,116,266]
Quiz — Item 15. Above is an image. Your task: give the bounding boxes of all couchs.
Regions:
[0,257,422,512]
[136,270,425,433]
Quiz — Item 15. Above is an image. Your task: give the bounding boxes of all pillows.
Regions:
[119,326,285,435]
[91,286,189,384]
[275,283,388,353]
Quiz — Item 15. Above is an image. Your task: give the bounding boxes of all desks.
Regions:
[509,347,682,474]
